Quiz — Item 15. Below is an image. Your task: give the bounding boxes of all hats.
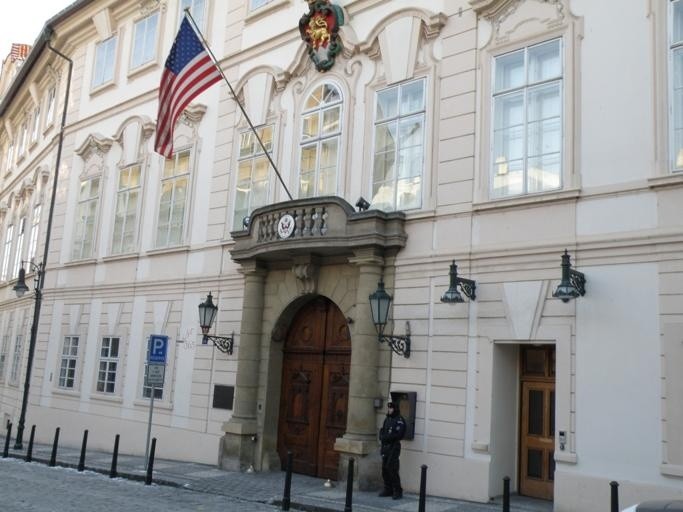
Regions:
[387,402,399,415]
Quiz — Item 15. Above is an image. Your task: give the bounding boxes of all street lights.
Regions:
[9,21,74,454]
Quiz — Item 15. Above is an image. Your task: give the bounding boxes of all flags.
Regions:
[152,16,223,160]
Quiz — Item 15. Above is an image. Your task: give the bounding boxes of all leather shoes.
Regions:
[391,491,402,500]
[378,489,391,497]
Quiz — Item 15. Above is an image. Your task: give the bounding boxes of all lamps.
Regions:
[366,277,410,359]
[551,249,588,304]
[243,217,250,230]
[356,196,369,212]
[439,257,476,305]
[197,292,233,356]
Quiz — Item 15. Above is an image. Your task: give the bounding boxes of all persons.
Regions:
[377,402,406,501]
